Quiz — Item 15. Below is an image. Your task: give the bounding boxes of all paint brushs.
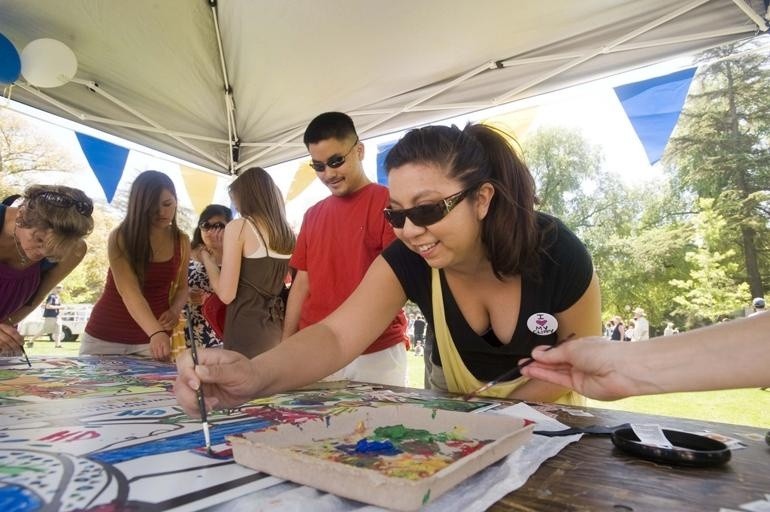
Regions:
[465,332,576,403]
[9,317,33,367]
[186,304,210,451]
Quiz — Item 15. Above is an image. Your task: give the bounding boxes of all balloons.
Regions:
[0,32,22,85]
[20,37,79,89]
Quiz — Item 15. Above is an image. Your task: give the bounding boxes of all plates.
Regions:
[609,426,732,465]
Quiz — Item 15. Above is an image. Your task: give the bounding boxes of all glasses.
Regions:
[308,143,360,173]
[382,186,476,234]
[30,190,98,218]
[198,220,224,232]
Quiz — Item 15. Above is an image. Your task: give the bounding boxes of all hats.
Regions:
[630,307,649,317]
[752,297,765,307]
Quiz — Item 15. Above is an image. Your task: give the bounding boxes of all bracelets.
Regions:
[8,316,14,325]
[149,331,167,338]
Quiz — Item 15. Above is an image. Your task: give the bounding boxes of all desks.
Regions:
[0,355,770,512]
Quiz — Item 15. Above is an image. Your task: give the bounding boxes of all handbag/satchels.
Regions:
[198,291,227,343]
[425,264,591,414]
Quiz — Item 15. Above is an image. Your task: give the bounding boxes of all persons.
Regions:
[605,307,650,341]
[0,185,95,357]
[171,126,604,424]
[27,285,66,349]
[406,313,416,351]
[280,111,411,386]
[663,321,678,336]
[751,297,767,314]
[515,308,770,402]
[78,170,191,359]
[413,339,425,357]
[179,168,297,360]
[413,315,425,347]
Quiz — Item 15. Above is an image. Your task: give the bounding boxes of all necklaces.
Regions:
[12,224,27,266]
